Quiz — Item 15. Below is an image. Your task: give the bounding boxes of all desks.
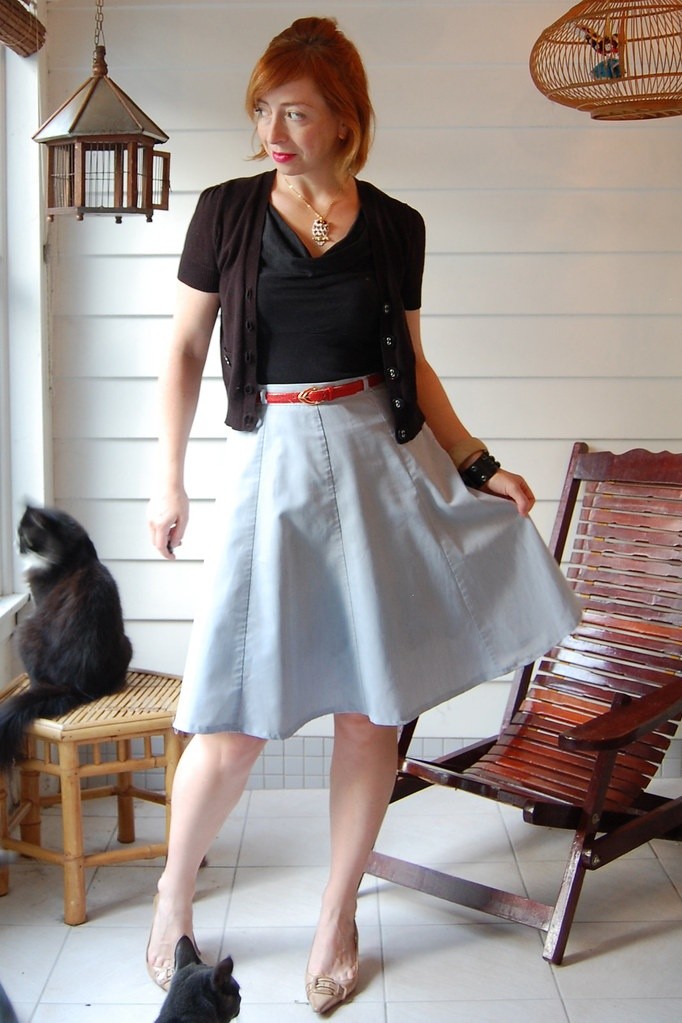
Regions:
[0,666,208,925]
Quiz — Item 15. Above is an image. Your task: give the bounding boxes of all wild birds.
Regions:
[574,23,622,57]
[590,58,627,79]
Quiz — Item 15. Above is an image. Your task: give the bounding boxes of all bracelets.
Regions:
[461,452,500,490]
[447,437,488,470]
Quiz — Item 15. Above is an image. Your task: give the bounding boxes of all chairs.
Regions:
[363,442,682,964]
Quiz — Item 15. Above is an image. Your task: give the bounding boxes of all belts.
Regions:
[256,373,386,404]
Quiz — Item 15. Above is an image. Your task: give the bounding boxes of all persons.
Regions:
[146,18,585,1013]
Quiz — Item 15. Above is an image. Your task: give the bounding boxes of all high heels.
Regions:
[146,893,202,992]
[305,918,359,1013]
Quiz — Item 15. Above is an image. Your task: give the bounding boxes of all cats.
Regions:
[0,506,135,773]
[0,935,241,1023]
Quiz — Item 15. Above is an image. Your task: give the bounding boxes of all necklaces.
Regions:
[285,174,351,246]
